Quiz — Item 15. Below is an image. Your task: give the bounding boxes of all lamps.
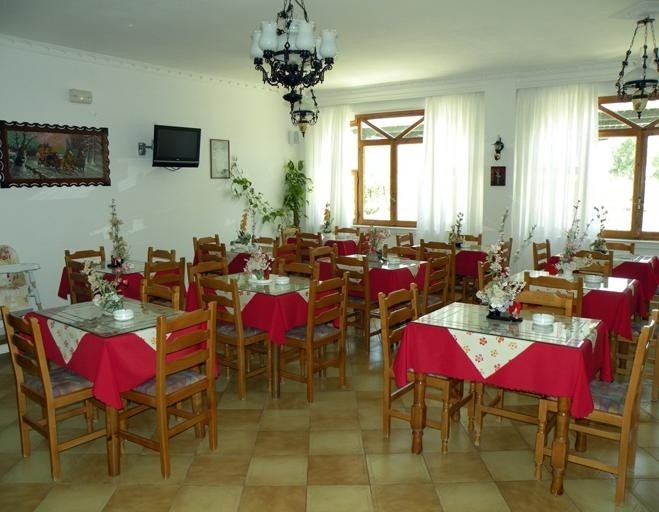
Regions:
[615,17,659,119]
[250,0,336,138]
[492,137,504,160]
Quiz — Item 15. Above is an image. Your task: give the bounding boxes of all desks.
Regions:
[17,292,209,478]
[393,299,616,496]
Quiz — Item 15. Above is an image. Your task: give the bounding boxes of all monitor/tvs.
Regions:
[152,125,201,167]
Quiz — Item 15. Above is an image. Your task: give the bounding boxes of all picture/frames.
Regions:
[0,120,111,188]
[210,139,230,178]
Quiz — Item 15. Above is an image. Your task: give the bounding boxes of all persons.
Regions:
[63,149,72,163]
[77,148,84,172]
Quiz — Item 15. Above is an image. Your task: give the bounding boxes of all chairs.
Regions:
[53,228,656,403]
[0,303,128,482]
[472,292,577,455]
[531,307,655,503]
[113,300,220,478]
[375,281,477,452]
